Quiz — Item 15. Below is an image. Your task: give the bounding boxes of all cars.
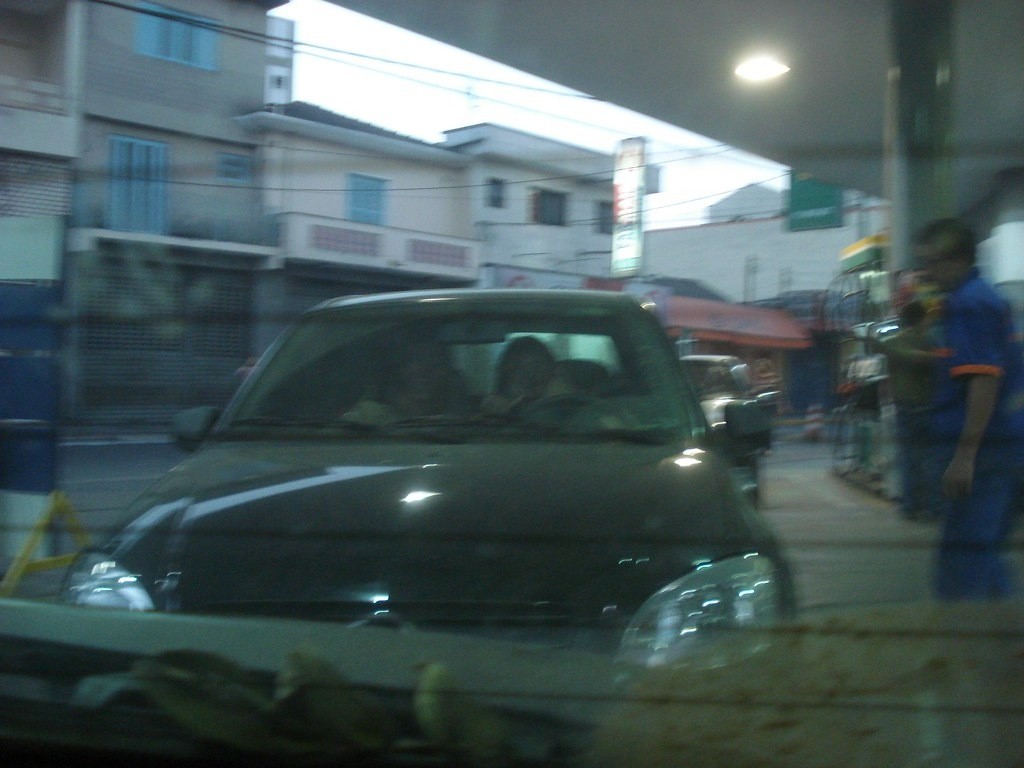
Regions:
[681,349,758,434]
[51,279,798,689]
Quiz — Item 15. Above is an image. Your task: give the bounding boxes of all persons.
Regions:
[888,304,940,520]
[913,219,1024,596]
[346,340,455,423]
[233,355,256,385]
[751,356,782,457]
[484,337,547,420]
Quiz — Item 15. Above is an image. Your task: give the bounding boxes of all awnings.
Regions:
[663,294,813,347]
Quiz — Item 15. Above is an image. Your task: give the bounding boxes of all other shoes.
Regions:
[900,502,934,518]
